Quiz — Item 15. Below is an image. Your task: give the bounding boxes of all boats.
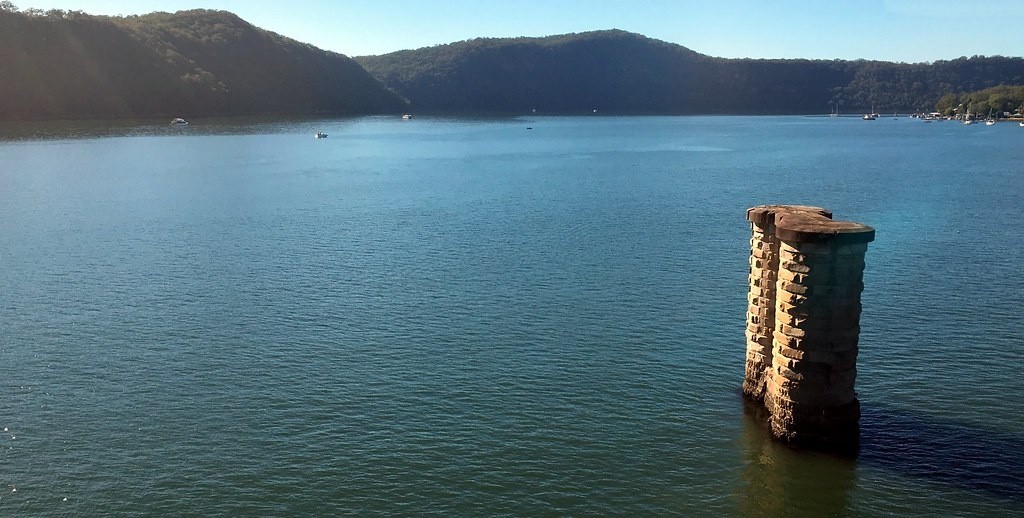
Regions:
[170,118,189,127]
[862,111,1024,130]
